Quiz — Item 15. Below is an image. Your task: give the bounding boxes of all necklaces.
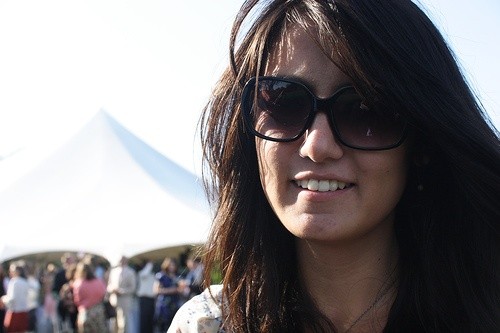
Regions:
[343,276,398,332]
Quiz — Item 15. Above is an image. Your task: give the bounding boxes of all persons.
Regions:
[0,246,213,333]
[167,0,500,333]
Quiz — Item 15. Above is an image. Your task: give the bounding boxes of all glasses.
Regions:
[240,71,412,153]
